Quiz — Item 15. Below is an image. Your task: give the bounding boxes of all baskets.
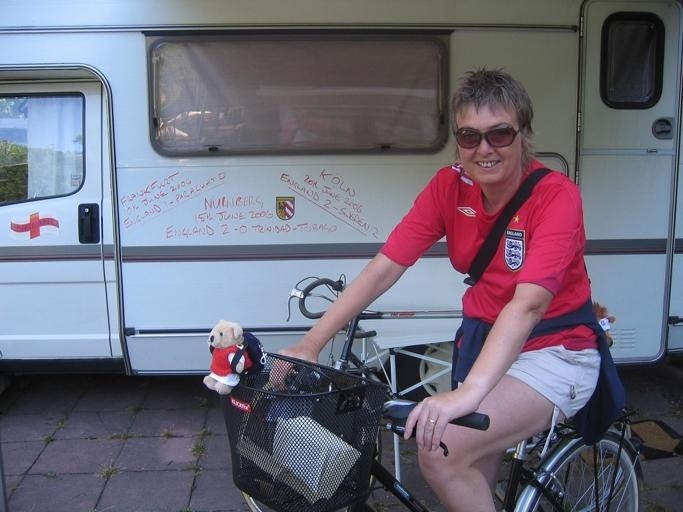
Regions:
[219,350,390,511]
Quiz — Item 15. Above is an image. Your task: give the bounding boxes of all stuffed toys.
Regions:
[200,318,252,396]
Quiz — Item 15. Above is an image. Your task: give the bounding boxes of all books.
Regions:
[231,413,363,504]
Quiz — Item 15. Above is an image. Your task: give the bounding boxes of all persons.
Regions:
[264,60,602,512]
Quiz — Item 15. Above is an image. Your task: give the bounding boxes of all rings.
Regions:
[428,417,436,424]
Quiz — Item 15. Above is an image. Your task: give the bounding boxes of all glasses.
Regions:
[452,122,528,150]
